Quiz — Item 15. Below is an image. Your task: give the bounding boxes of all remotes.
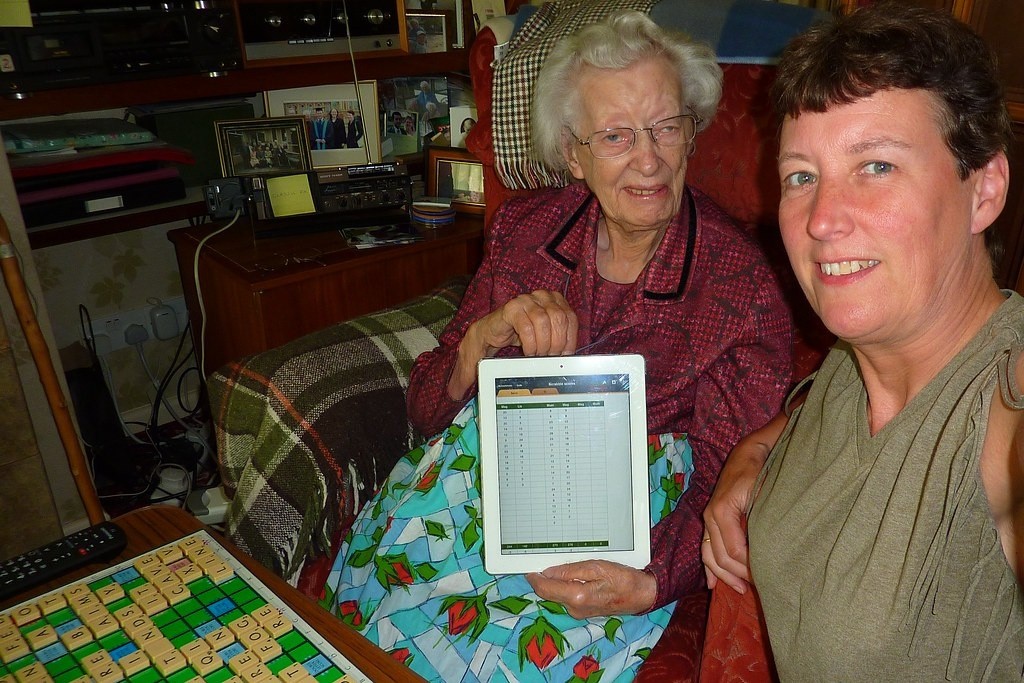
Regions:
[0,521,126,603]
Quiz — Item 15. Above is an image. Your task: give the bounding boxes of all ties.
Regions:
[397,130,399,134]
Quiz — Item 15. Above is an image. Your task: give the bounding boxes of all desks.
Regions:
[0,504,431,682]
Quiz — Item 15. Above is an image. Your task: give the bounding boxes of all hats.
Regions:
[314,106,326,112]
[417,30,425,36]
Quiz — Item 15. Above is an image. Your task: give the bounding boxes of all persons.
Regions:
[317,9,796,683]
[289,107,363,150]
[700,4,1024,683]
[461,120,476,140]
[387,112,415,136]
[242,139,288,170]
[415,81,441,121]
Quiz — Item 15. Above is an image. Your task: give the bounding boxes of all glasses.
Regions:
[566,104,703,159]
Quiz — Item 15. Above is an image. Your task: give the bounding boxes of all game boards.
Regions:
[0,528,377,683]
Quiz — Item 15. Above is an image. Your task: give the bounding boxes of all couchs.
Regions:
[208,62,1024,683]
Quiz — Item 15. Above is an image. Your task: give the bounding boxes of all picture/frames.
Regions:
[213,115,313,178]
[405,9,451,54]
[423,145,486,214]
[263,79,382,169]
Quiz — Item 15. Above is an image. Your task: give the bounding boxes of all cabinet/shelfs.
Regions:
[166,212,484,377]
[0,48,470,249]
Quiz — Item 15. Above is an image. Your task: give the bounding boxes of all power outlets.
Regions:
[78,295,189,356]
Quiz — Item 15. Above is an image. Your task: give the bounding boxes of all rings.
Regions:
[702,538,712,543]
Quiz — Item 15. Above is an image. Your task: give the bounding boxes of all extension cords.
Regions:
[149,426,210,506]
[193,485,231,525]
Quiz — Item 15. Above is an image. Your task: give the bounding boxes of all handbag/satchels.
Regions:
[55,304,128,482]
[355,121,365,147]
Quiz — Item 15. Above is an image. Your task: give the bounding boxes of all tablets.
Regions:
[477,353,650,576]
